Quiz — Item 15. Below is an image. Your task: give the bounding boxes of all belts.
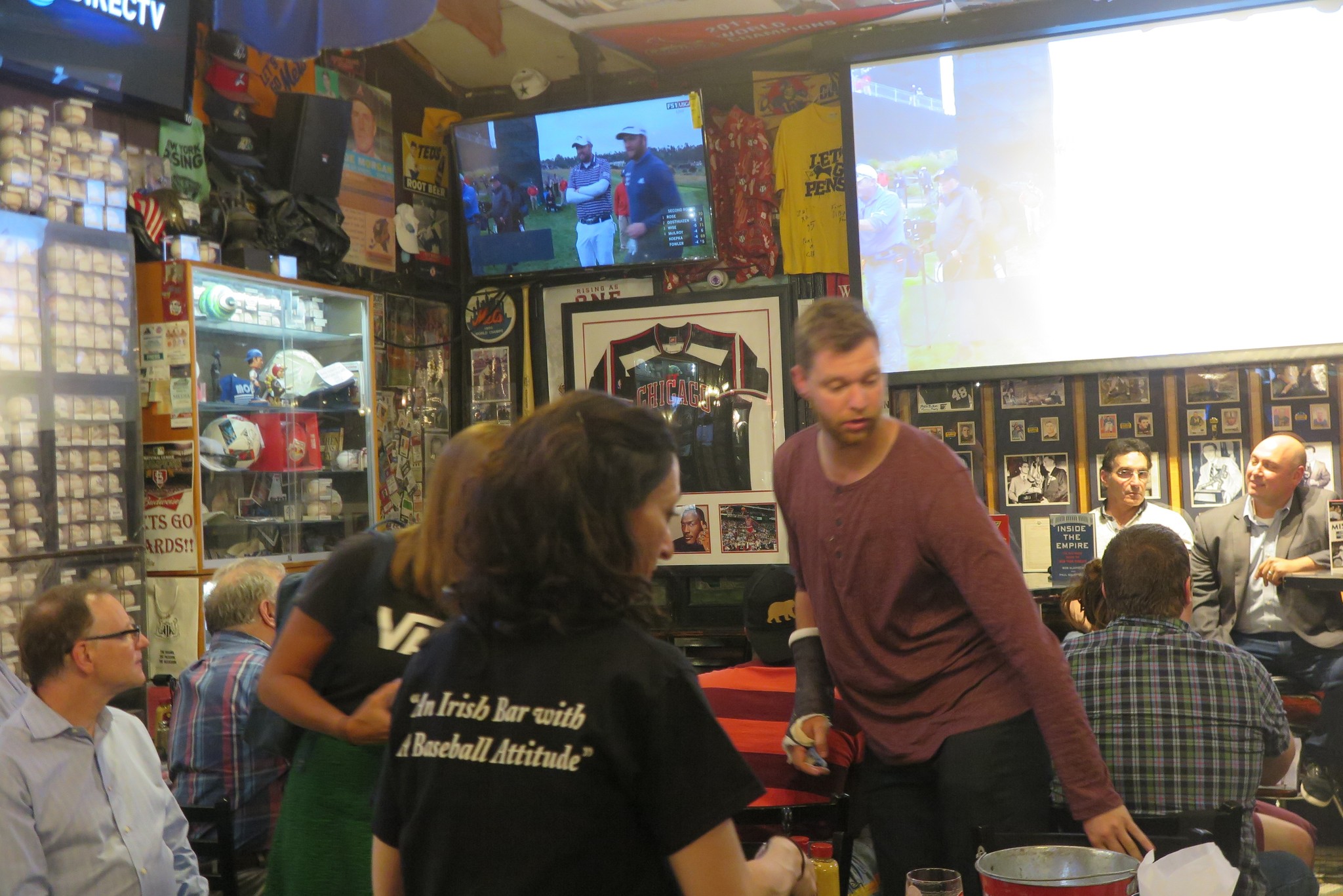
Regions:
[579,213,611,226]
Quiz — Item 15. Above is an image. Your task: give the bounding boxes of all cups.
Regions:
[906,868,964,896]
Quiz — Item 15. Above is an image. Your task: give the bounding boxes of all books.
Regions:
[1049,513,1098,585]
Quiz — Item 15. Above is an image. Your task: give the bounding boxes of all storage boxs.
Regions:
[0,91,150,686]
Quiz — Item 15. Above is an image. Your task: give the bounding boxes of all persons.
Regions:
[1079,438,1193,571]
[671,506,711,553]
[210,344,292,408]
[741,506,761,551]
[1189,432,1343,806]
[954,362,1331,504]
[1073,560,1317,870]
[255,421,517,896]
[369,389,818,896]
[167,556,289,896]
[0,581,209,896]
[771,294,1153,895]
[1052,524,1320,896]
[461,123,686,268]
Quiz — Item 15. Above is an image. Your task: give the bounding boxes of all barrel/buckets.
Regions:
[975,845,1141,896]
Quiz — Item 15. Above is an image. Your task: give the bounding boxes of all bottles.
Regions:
[156,721,170,752]
[807,842,840,896]
[791,836,809,857]
[156,697,172,726]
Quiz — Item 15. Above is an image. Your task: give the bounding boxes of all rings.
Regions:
[697,540,703,545]
[1268,570,1273,575]
[702,530,707,535]
[700,536,705,539]
[702,524,706,529]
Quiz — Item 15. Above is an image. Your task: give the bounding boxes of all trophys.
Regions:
[1194,465,1229,503]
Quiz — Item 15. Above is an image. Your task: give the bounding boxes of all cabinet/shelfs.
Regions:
[0,209,377,773]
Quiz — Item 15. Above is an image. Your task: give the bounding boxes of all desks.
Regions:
[1279,565,1343,632]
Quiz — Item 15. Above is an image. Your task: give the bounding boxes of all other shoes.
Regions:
[1302,759,1339,807]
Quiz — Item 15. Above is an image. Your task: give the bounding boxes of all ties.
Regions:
[1210,463,1214,477]
[1023,476,1026,484]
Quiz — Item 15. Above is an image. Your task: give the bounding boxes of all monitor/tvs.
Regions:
[449,89,719,290]
[0,0,199,127]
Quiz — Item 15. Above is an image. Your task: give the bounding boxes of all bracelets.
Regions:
[787,837,806,884]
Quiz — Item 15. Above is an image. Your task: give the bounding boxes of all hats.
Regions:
[489,173,501,181]
[620,169,627,176]
[459,173,466,181]
[615,124,648,141]
[572,135,592,146]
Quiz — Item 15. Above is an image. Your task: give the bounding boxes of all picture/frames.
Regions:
[1271,404,1293,433]
[1040,417,1060,441]
[1184,364,1240,404]
[1309,403,1332,431]
[1300,440,1335,493]
[1009,418,1026,442]
[953,451,974,490]
[957,421,976,446]
[1187,438,1245,509]
[1097,369,1151,407]
[916,375,974,413]
[919,425,944,443]
[1133,412,1154,438]
[1096,451,1161,501]
[1269,357,1330,401]
[1004,451,1070,507]
[1221,407,1242,435]
[1000,375,1067,410]
[1186,408,1208,436]
[1098,413,1119,440]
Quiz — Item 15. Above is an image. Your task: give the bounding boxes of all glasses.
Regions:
[1112,470,1150,478]
[72,616,143,644]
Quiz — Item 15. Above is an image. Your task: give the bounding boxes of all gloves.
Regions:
[626,237,638,255]
